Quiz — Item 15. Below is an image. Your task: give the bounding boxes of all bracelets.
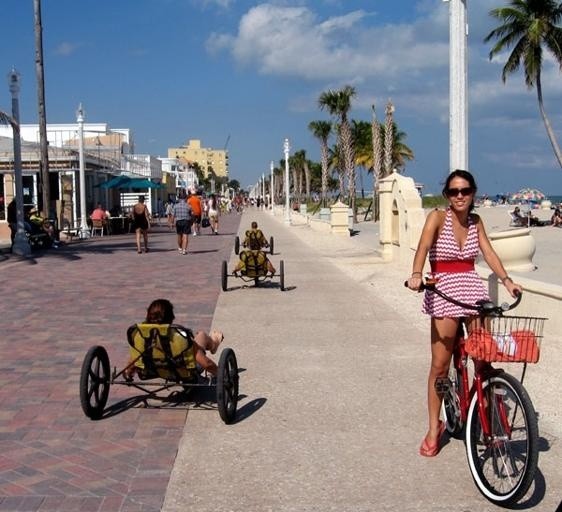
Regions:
[502,276,513,285]
[411,272,422,277]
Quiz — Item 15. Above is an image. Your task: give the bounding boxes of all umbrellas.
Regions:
[94,176,166,193]
[509,187,547,227]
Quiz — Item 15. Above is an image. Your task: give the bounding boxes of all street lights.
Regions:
[8,63,31,260]
[282,138,291,226]
[270,160,276,216]
[75,102,90,240]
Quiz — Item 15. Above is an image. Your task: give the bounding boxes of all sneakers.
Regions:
[178,248,188,255]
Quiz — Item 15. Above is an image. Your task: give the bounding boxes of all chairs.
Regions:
[89,219,111,237]
[65,219,83,241]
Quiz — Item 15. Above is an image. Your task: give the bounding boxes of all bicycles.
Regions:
[404,277,549,506]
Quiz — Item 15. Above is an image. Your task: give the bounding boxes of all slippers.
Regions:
[419,419,446,458]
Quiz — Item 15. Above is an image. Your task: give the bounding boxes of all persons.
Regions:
[170,193,193,256]
[91,204,107,227]
[186,190,201,237]
[133,195,152,254]
[231,241,276,275]
[165,198,173,221]
[30,208,43,223]
[120,299,223,384]
[481,194,561,227]
[405,168,527,454]
[240,221,268,248]
[204,194,221,235]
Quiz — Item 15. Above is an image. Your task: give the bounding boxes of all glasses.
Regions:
[447,187,474,198]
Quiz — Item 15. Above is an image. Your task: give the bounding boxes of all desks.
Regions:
[110,217,128,233]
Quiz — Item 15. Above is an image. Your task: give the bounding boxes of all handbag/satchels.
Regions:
[201,218,211,228]
